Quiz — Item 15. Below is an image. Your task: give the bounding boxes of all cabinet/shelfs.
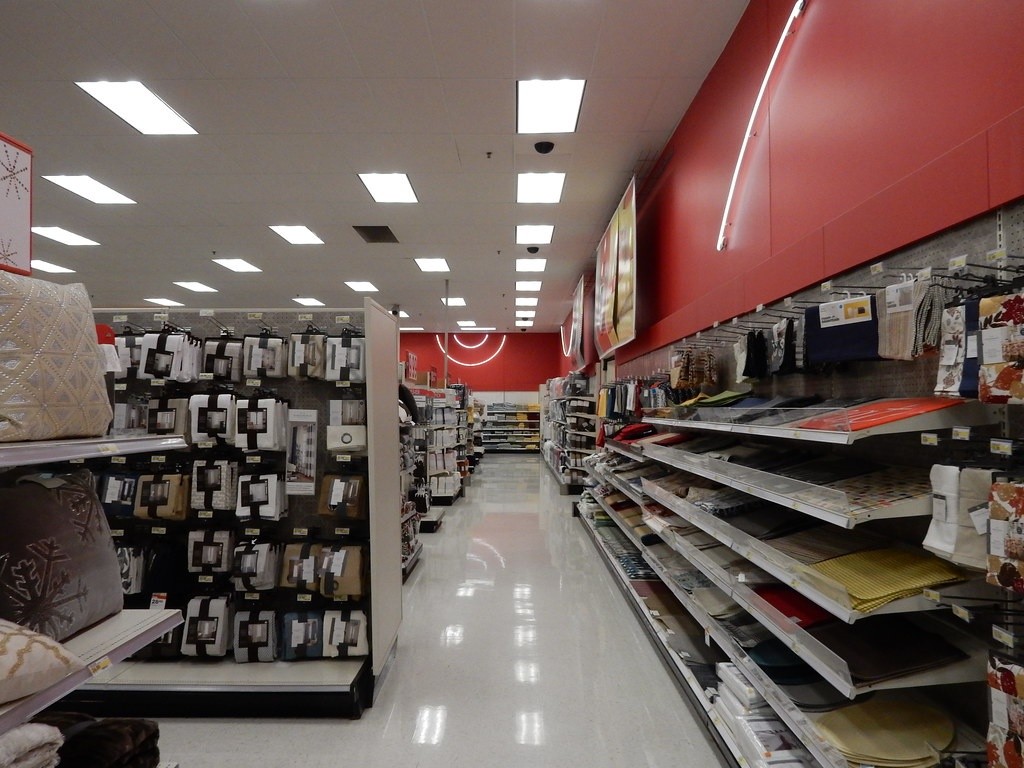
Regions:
[78,246,1024,768]
[0,433,190,768]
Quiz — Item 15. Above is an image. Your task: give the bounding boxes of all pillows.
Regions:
[0,468,124,644]
[0,618,86,705]
[0,270,114,443]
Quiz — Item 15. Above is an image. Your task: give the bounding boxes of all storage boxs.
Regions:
[0,132,34,277]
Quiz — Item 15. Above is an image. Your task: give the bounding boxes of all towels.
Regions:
[577,277,1024,768]
